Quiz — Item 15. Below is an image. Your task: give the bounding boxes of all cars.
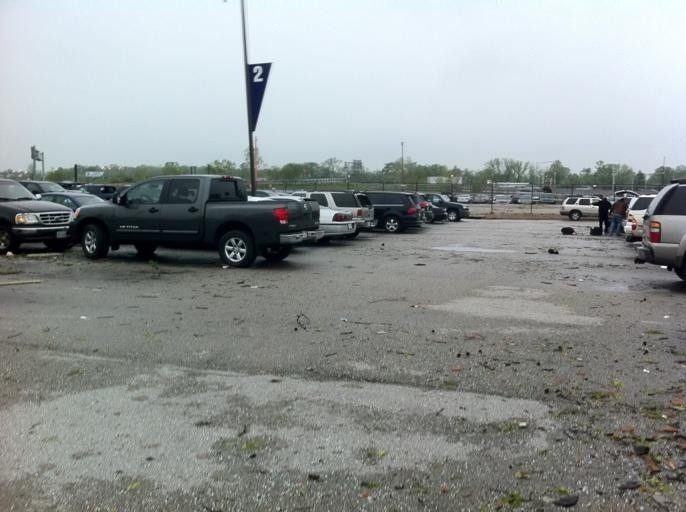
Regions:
[455,191,583,206]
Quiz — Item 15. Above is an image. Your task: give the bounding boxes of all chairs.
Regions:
[172,186,191,203]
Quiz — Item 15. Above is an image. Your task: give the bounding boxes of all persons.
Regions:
[592,195,631,237]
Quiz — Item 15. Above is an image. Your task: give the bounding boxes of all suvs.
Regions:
[558,195,612,221]
[609,177,686,287]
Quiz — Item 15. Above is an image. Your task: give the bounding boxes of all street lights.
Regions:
[400,140,406,191]
